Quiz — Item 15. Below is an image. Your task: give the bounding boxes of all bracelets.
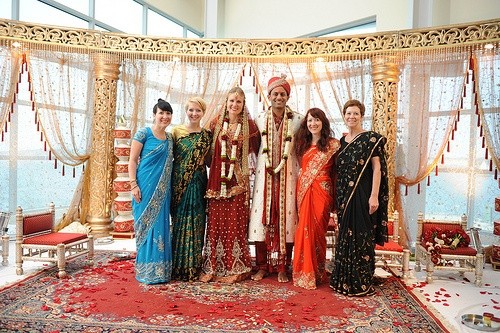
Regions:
[129,185,137,190]
[129,179,137,185]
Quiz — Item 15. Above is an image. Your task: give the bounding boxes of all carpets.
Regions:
[0,250,449,333]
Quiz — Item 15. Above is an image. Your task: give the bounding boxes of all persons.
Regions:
[171,96,213,283]
[329,99,389,297]
[248,72,306,283]
[199,86,262,284]
[291,107,341,290]
[128,97,175,285]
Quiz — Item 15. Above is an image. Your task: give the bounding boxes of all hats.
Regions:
[268,77,290,95]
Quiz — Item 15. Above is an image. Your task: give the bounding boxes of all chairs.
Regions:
[415,211,485,285]
[323,213,341,262]
[372,209,410,277]
[14,202,94,278]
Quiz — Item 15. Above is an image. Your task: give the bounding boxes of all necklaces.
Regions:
[229,121,237,127]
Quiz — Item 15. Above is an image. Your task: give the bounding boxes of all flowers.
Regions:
[423,225,472,251]
